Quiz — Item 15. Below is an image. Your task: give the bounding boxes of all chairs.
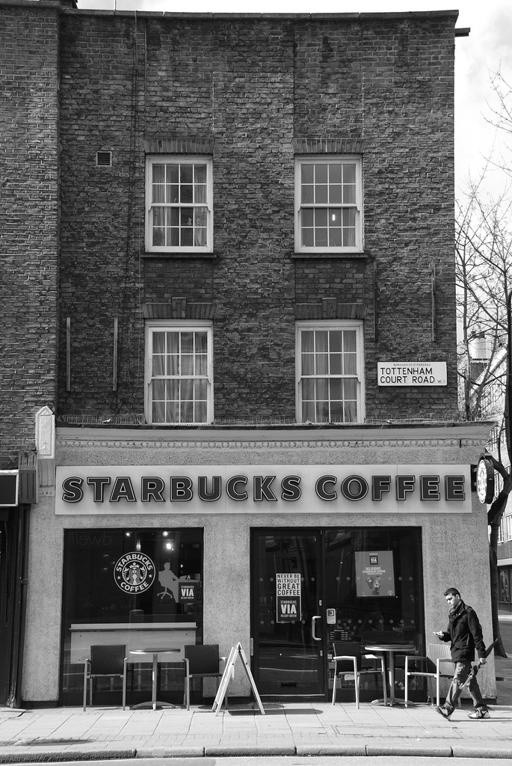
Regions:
[183,644,228,711]
[405,643,461,709]
[332,641,387,710]
[83,645,134,710]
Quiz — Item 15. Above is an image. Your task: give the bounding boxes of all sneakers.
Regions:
[467,710,490,719]
[436,706,451,720]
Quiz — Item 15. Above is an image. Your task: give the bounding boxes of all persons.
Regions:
[433,588,490,721]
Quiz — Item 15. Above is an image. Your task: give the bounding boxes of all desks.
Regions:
[365,644,416,707]
[128,648,181,710]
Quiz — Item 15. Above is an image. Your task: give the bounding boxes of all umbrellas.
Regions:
[459,638,499,690]
